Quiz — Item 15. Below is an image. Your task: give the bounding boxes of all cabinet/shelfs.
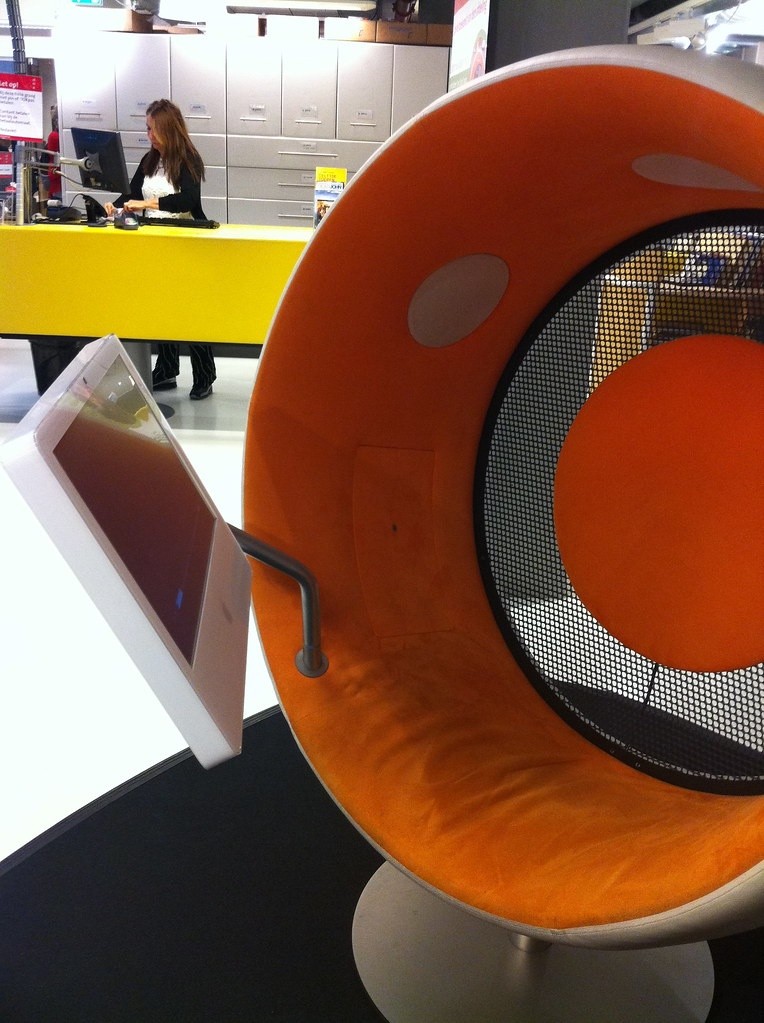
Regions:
[46,22,450,229]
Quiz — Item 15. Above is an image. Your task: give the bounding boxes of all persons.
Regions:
[100,95,218,402]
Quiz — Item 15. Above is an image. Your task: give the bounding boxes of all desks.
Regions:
[1,216,314,360]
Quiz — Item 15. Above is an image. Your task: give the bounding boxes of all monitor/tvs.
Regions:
[72,126,130,194]
[0,328,252,767]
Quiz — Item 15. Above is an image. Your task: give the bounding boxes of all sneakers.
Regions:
[148,371,177,392]
[188,382,213,401]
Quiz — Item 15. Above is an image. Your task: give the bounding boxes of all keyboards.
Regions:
[136,217,219,228]
